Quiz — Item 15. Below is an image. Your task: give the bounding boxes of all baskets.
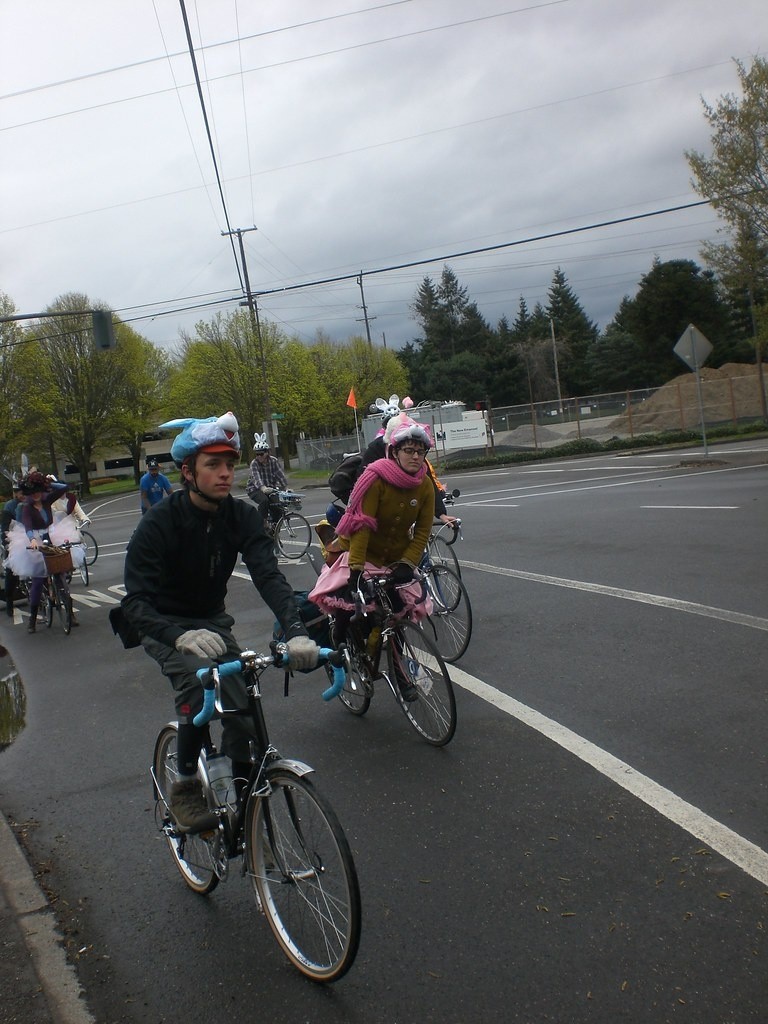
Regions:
[41,552,72,574]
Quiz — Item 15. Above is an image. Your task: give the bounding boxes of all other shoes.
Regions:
[389,669,418,702]
[6,600,13,617]
[71,616,79,626]
[27,615,36,633]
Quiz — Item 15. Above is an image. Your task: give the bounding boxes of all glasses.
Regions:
[255,451,267,457]
[396,446,427,457]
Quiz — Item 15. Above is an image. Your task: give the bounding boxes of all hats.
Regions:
[252,432,270,451]
[384,414,436,447]
[182,426,241,465]
[148,460,158,470]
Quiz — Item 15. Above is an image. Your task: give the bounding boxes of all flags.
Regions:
[346,388,356,407]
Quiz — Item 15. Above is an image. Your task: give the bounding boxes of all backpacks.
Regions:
[271,591,330,675]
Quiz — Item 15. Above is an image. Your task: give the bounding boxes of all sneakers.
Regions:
[240,828,274,876]
[168,782,220,833]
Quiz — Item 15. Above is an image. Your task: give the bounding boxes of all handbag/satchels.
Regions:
[109,606,142,649]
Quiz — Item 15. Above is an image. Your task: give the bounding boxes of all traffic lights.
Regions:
[474,400,487,411]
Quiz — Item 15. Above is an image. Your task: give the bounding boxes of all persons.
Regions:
[0,471,91,635]
[123,409,320,877]
[309,415,457,703]
[246,440,290,547]
[140,458,173,516]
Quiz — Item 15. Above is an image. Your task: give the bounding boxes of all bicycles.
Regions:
[148,638,364,985]
[261,484,313,561]
[8,520,99,636]
[314,481,474,749]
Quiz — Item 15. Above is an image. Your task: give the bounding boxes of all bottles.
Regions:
[204,742,237,807]
[365,627,382,656]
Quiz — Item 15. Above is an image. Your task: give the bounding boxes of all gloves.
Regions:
[348,569,364,591]
[175,629,228,659]
[285,635,319,673]
[390,564,413,585]
